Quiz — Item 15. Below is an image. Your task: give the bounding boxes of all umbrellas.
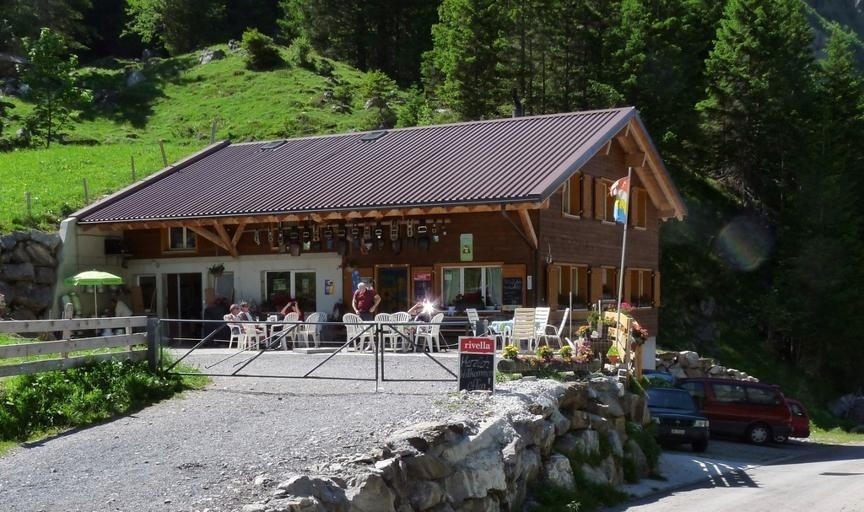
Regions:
[63,268,121,335]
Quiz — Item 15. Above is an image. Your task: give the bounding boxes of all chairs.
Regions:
[466,307,570,350]
[342,312,445,353]
[224,312,329,351]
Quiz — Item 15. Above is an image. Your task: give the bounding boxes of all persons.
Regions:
[107,298,134,335]
[223,304,263,349]
[350,282,380,352]
[237,301,275,351]
[405,297,440,352]
[274,298,303,351]
[100,306,115,317]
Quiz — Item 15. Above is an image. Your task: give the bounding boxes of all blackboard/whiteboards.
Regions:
[458,352,495,390]
[502,277,522,305]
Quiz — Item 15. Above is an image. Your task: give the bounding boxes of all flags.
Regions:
[610,176,629,224]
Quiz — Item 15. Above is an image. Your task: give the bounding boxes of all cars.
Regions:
[646,376,811,452]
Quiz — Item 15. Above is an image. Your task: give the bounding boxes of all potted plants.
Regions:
[504,312,620,364]
